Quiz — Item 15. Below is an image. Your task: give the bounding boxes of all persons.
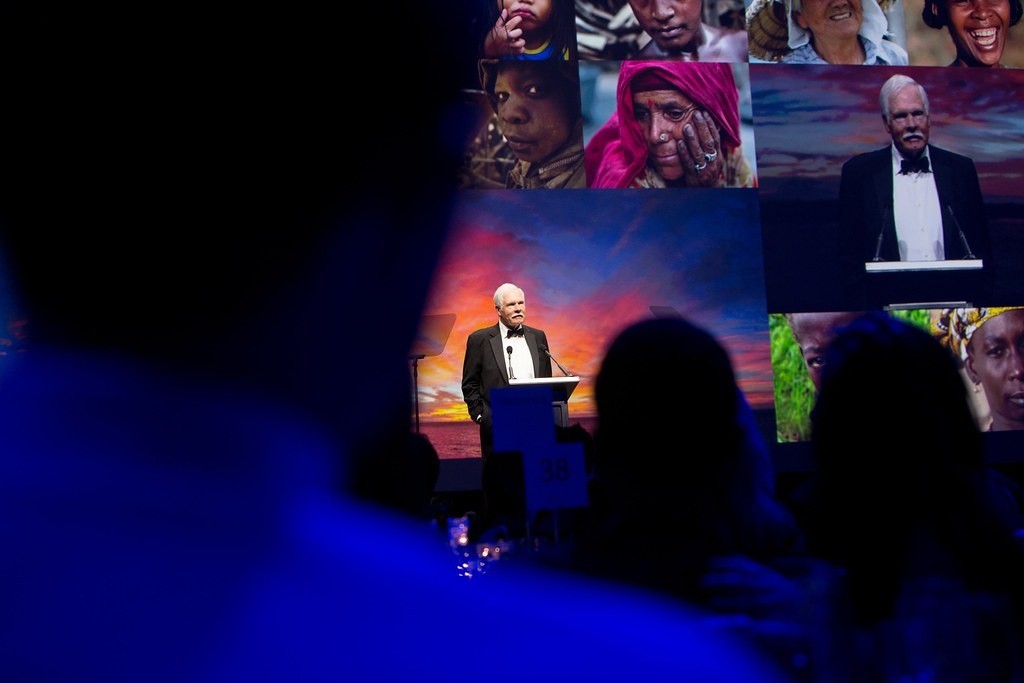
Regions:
[783,310,864,392]
[837,74,986,309]
[747,0,908,65]
[584,61,758,189]
[628,0,748,63]
[922,0,1024,70]
[464,62,585,187]
[930,307,1024,431]
[479,0,571,61]
[0,0,1024,683]
[462,283,552,464]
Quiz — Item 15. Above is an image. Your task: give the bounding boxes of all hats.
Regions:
[745,0,899,62]
[923,0,1023,29]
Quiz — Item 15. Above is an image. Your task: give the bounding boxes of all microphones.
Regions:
[944,199,977,261]
[876,199,892,261]
[539,344,571,376]
[507,346,516,379]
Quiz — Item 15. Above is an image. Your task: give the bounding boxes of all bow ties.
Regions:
[897,156,934,175]
[507,328,524,339]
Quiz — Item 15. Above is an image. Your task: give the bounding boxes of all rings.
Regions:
[695,162,706,170]
[703,149,717,161]
